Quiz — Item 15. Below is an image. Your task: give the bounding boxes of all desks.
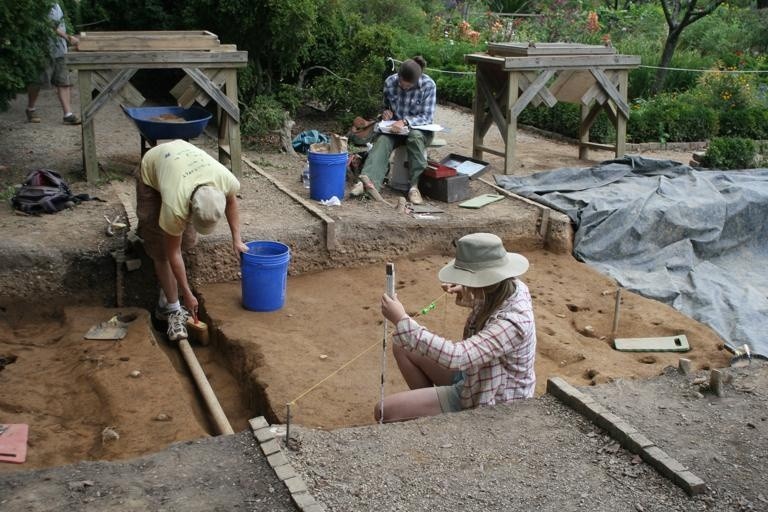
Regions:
[464,42,642,177]
[63,28,251,188]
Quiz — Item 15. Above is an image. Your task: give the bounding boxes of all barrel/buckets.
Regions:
[307,148,348,201]
[239,241,290,312]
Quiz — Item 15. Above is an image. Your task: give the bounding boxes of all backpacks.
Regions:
[13,168,105,216]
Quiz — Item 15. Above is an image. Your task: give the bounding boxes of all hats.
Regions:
[191,183,228,236]
[436,231,530,289]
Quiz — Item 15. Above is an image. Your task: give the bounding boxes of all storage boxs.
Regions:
[418,152,492,203]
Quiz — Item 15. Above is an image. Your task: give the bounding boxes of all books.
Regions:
[454,160,486,181]
[443,159,461,170]
[373,120,445,135]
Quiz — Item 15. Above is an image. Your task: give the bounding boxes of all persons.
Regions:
[26,1,81,124]
[133,138,249,340]
[349,55,437,205]
[374,231,537,424]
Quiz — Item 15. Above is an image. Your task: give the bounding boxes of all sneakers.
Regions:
[62,112,82,125]
[25,106,40,123]
[407,187,424,208]
[349,182,364,198]
[155,302,188,340]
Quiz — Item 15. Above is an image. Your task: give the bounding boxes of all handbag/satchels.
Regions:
[352,115,377,145]
